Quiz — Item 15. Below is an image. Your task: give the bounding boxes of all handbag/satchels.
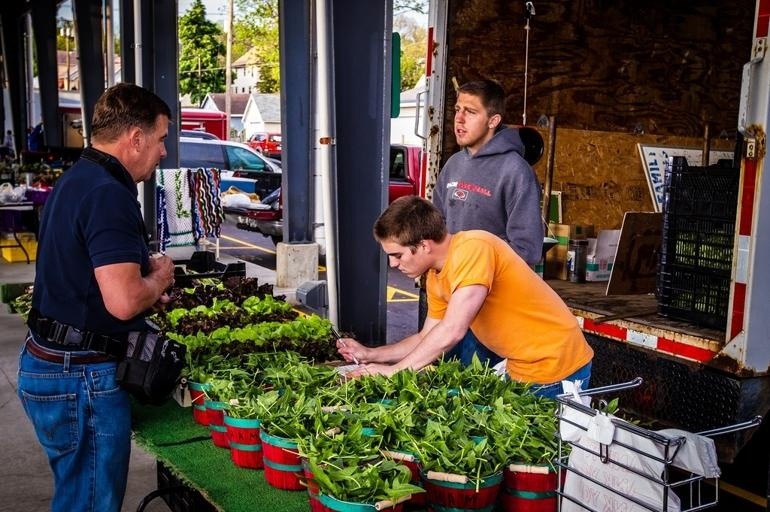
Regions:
[24,308,187,405]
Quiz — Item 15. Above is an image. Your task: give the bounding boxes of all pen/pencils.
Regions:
[329,327,360,368]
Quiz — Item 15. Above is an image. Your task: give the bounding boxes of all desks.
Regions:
[0,202,35,264]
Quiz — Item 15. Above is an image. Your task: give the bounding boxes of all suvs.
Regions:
[245,133,286,156]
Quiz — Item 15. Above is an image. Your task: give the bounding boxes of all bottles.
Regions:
[567,238,589,284]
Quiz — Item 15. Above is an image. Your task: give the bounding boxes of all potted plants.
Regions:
[184,355,224,427]
[259,381,324,489]
[293,418,426,511]
[224,376,283,469]
[493,387,657,512]
[414,399,505,512]
[204,363,242,447]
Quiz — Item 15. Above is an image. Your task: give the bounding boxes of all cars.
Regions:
[388,142,421,203]
[178,139,283,182]
[179,130,220,139]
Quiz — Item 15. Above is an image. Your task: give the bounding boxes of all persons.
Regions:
[13,81,175,512]
[427,77,545,373]
[336,194,596,407]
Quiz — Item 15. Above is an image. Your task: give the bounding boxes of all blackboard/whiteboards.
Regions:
[540,190,562,225]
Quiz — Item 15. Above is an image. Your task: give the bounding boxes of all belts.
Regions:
[23,335,110,364]
